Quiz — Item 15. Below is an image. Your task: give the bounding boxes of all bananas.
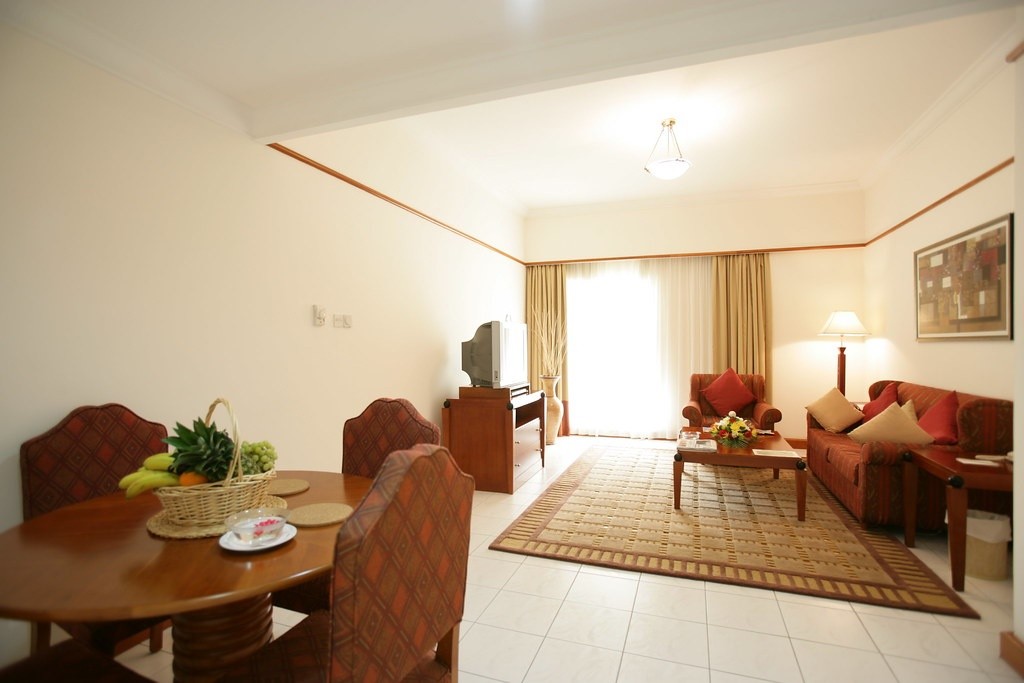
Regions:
[118,452,180,498]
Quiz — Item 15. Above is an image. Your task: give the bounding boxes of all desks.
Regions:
[673,427,808,523]
[900,447,1013,593]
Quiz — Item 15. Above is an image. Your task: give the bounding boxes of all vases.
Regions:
[536,374,563,446]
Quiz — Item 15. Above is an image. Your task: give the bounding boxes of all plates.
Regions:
[219,523,298,552]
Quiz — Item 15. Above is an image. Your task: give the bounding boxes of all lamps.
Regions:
[817,308,871,398]
[643,117,690,180]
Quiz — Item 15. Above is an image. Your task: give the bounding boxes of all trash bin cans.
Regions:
[944,509,1012,582]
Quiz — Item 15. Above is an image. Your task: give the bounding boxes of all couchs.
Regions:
[803,379,1013,530]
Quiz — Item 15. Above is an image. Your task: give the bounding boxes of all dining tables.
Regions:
[0,468,372,683]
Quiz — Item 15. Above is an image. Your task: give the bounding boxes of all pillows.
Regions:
[899,398,917,424]
[857,383,900,422]
[805,388,862,435]
[918,390,961,445]
[700,366,757,418]
[845,404,932,446]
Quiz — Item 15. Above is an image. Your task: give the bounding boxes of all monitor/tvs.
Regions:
[461,319,528,389]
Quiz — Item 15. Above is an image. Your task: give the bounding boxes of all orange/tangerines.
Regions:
[180,471,206,486]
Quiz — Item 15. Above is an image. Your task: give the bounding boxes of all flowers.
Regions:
[702,411,758,445]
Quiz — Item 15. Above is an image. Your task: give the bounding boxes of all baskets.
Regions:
[150,398,278,526]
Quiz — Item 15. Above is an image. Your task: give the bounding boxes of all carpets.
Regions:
[488,440,978,619]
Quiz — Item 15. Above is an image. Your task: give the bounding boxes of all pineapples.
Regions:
[161,416,262,480]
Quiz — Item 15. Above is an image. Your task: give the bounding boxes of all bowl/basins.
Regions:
[682,431,701,448]
[224,507,291,547]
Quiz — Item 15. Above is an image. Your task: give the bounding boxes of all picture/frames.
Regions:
[913,212,1014,341]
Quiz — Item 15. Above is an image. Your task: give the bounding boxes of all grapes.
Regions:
[241,440,278,474]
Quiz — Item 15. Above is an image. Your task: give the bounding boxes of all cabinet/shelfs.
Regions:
[443,382,545,495]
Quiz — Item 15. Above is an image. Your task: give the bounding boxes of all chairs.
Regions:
[682,373,783,479]
[0,398,475,683]
